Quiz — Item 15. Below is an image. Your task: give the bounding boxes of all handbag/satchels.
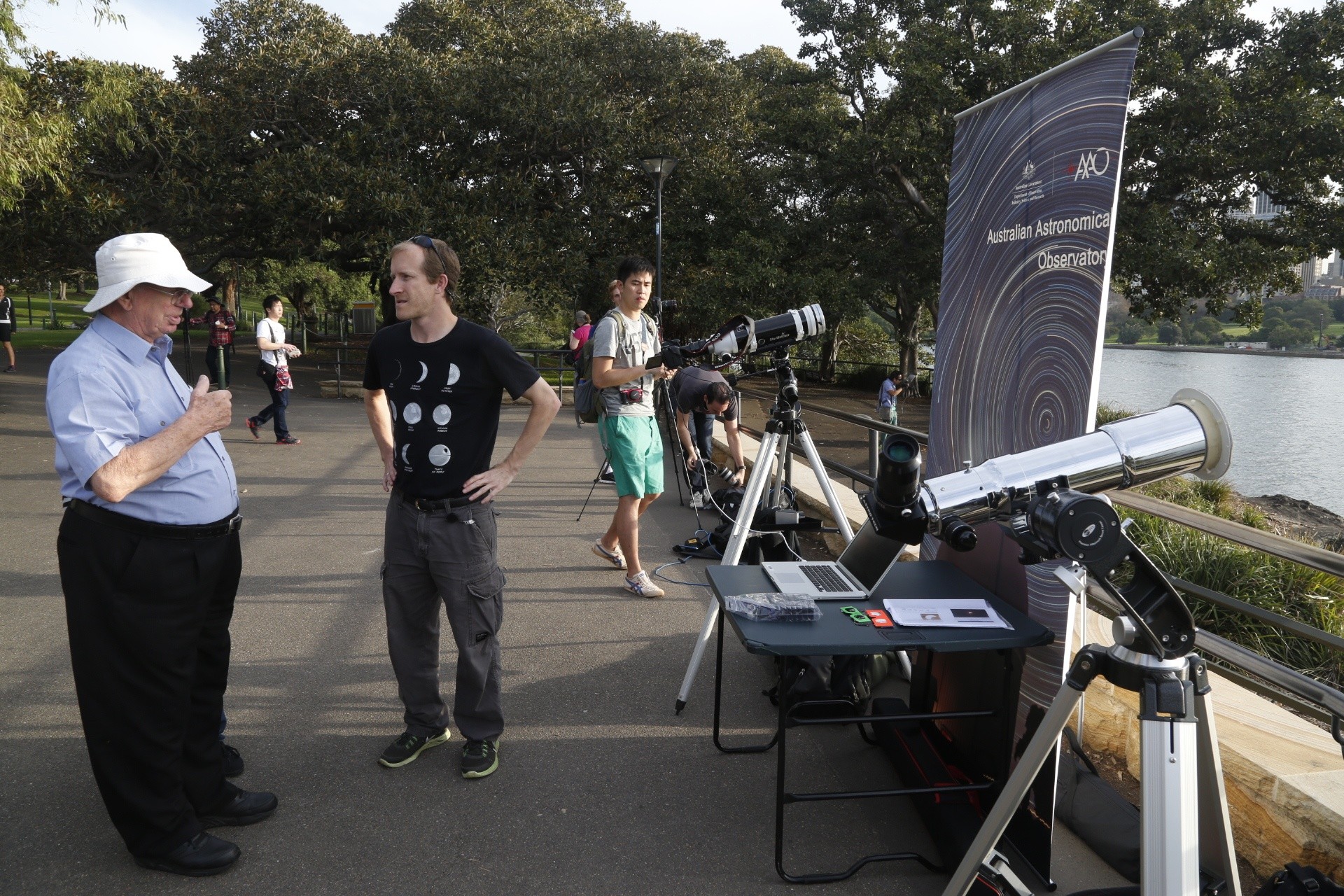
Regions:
[256,359,277,382]
[878,407,892,421]
[565,351,575,365]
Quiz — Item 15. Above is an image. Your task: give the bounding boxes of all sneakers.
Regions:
[379,726,452,767]
[195,778,279,829]
[460,736,499,778]
[133,830,243,876]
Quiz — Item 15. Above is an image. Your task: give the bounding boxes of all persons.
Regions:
[361,236,562,778]
[47,234,281,876]
[589,280,621,485]
[569,310,596,384]
[669,367,747,509]
[190,296,236,389]
[0,284,17,372]
[879,370,909,449]
[590,256,677,597]
[246,295,301,446]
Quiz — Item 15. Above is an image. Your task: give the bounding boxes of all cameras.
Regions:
[648,296,677,314]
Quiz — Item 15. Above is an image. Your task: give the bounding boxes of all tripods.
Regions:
[576,376,703,531]
[673,347,855,718]
[943,486,1244,896]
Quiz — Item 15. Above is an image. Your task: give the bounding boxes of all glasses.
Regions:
[898,378,902,381]
[407,235,447,273]
[141,284,194,305]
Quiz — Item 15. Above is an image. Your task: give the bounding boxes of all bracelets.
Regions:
[225,326,227,329]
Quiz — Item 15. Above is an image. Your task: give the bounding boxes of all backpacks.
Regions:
[572,309,654,424]
[206,310,237,354]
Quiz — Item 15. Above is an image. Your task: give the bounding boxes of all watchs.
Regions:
[738,466,746,470]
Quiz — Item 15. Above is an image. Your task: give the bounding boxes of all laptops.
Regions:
[761,517,907,601]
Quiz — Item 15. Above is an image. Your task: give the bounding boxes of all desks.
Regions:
[705,563,1053,882]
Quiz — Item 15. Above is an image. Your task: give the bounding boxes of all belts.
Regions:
[69,499,245,538]
[392,486,490,512]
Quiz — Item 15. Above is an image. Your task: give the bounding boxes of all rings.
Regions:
[387,473,389,475]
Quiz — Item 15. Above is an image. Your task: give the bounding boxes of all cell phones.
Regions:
[570,329,575,334]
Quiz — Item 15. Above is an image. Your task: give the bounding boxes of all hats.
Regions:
[81,233,213,313]
[205,297,225,308]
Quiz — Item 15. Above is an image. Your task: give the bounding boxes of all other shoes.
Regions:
[591,539,628,569]
[210,379,218,385]
[246,417,260,439]
[623,569,664,597]
[4,365,17,372]
[225,384,230,389]
[276,434,301,445]
[689,491,704,510]
[703,489,710,503]
